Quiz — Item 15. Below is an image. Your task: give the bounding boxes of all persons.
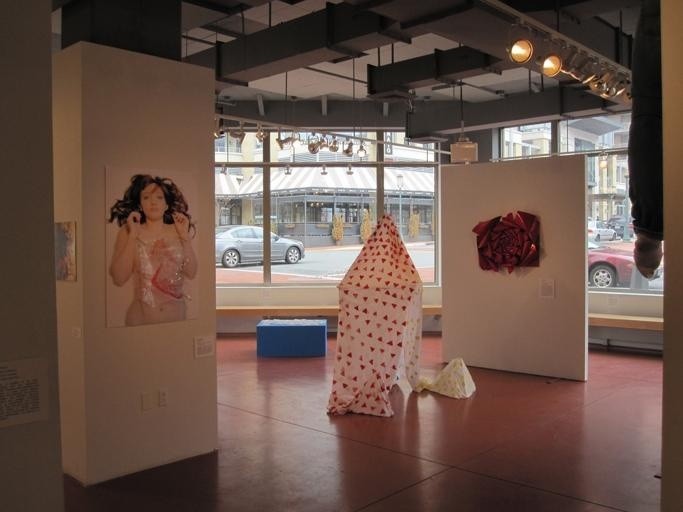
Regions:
[621,2,664,281]
[104,170,195,328]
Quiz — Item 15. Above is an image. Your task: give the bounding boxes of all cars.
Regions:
[213,223,305,268]
[585,215,663,288]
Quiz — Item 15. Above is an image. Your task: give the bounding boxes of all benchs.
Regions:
[257,318,328,358]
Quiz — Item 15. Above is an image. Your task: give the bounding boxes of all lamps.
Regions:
[506,21,631,97]
[450,79,479,163]
[214,119,367,159]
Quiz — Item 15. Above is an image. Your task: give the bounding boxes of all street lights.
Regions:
[622,174,629,242]
[399,173,403,242]
